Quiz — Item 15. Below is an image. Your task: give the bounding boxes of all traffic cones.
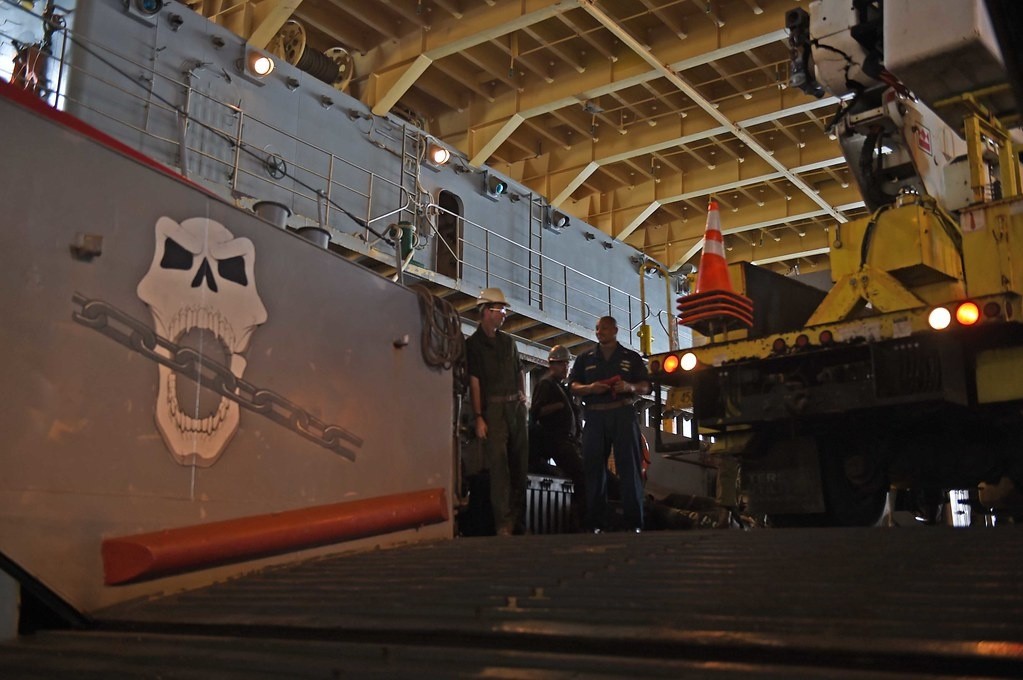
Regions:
[676,201,754,336]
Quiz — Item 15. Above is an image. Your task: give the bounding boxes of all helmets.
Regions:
[476,287,510,307]
[548,345,572,360]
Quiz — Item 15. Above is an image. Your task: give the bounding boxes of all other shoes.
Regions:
[497,522,514,536]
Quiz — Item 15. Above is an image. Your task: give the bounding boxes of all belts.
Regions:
[587,398,633,410]
[487,393,519,403]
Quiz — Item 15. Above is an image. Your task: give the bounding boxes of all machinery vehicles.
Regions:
[647,0,1023,527]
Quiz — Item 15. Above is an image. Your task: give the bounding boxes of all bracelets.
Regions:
[631,385,635,391]
[474,414,481,419]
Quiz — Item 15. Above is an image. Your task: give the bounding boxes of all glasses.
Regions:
[490,307,506,315]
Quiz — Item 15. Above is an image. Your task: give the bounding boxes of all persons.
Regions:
[567,316,654,534]
[465,288,529,535]
[717,456,741,528]
[529,344,584,500]
